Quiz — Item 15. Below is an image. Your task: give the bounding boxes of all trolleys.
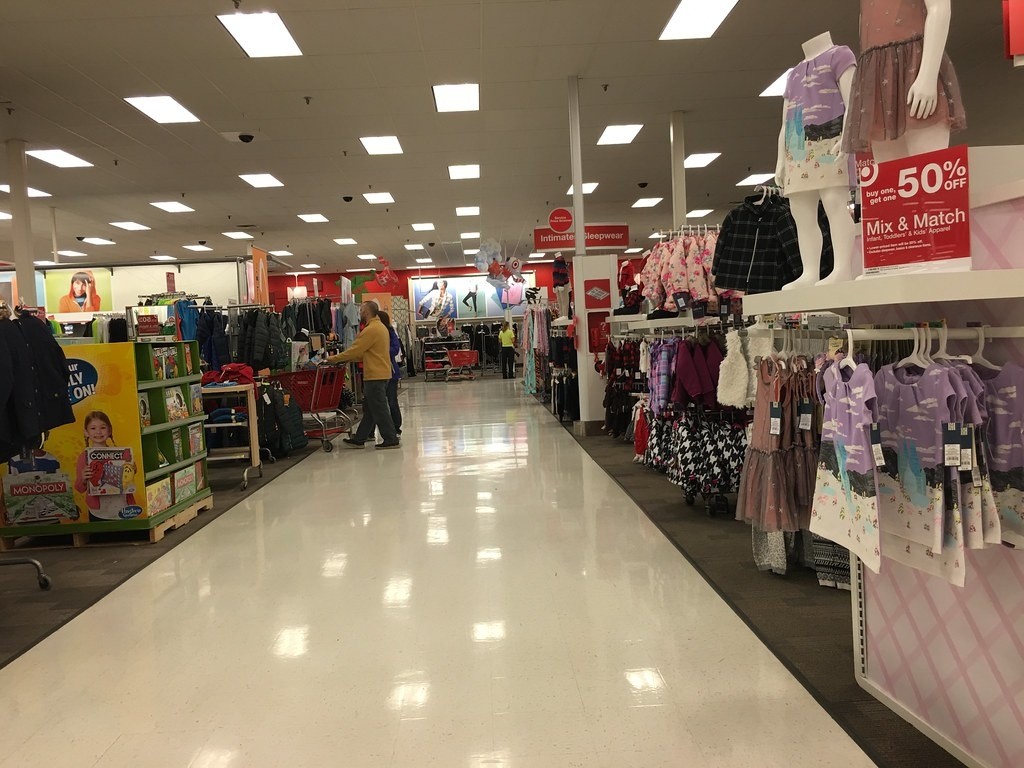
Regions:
[442,347,478,382]
[265,360,356,452]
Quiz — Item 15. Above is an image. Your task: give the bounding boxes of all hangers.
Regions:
[895,326,926,369]
[96,291,340,320]
[930,323,973,365]
[419,320,501,334]
[607,322,734,422]
[659,223,720,242]
[904,328,931,365]
[0,301,19,321]
[971,327,1002,371]
[755,314,863,374]
[752,185,784,205]
[923,327,935,363]
[525,298,576,378]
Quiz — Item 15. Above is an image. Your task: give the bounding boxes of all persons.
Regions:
[840,0,967,164]
[417,280,454,320]
[774,30,858,290]
[324,300,402,449]
[462,278,478,317]
[7,430,61,474]
[552,251,572,321]
[498,321,515,379]
[350,310,402,441]
[617,260,634,307]
[58,271,101,313]
[74,410,137,522]
[638,249,652,300]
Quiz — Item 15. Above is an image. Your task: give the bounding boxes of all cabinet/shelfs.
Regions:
[423,336,472,382]
[202,381,261,492]
[130,340,215,543]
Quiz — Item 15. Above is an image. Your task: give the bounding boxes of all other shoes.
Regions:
[343,438,364,449]
[348,433,375,441]
[396,434,401,439]
[374,443,400,449]
